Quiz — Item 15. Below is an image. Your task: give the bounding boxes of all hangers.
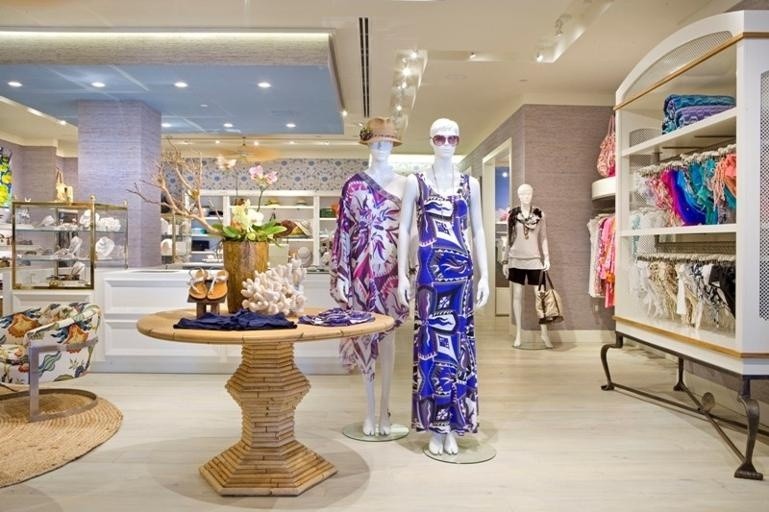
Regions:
[642,143,737,177]
[638,246,735,265]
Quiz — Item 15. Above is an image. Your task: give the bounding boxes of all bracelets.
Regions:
[501,260,508,265]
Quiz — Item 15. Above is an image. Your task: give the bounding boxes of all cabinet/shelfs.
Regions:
[600,11,769,481]
[184,190,342,274]
[12,203,129,292]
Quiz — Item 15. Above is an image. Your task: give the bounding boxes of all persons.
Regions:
[501,183,556,349]
[330,116,421,437]
[396,117,491,456]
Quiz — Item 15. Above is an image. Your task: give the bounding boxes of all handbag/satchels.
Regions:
[56,181,73,203]
[535,289,564,324]
[596,132,615,177]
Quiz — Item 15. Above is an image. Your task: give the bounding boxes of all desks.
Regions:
[137,305,395,499]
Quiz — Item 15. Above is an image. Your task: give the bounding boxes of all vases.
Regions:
[222,240,269,314]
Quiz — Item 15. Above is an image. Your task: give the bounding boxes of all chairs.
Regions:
[0,302,102,423]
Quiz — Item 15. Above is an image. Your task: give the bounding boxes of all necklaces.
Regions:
[431,163,456,230]
[518,206,534,240]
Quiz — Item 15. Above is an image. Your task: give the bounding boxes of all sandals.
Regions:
[186,267,208,299]
[207,270,230,300]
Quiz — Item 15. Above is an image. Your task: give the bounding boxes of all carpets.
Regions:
[0,384,124,489]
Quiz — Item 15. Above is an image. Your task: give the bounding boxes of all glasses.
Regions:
[429,136,459,146]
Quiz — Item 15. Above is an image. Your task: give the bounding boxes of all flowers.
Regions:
[211,157,289,249]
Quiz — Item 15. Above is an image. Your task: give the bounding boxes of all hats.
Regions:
[296,247,312,267]
[264,199,280,206]
[280,220,313,238]
[230,198,244,205]
[294,199,308,205]
[357,115,402,147]
